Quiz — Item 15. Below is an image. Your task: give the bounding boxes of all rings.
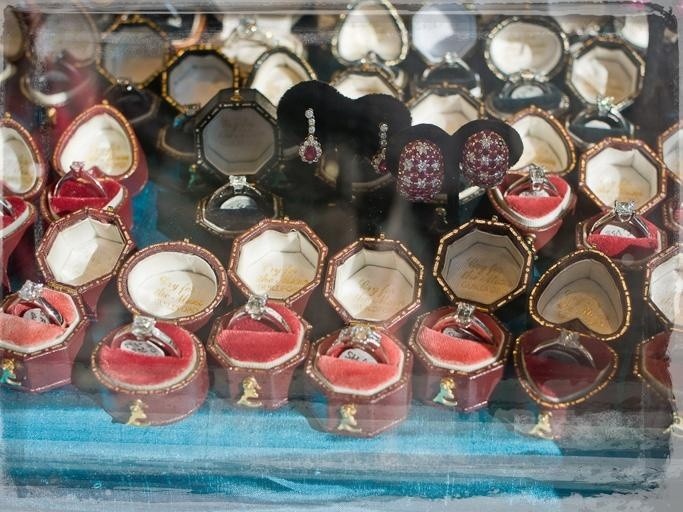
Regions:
[225,293,291,331]
[530,328,596,371]
[1,6,647,235]
[323,324,393,364]
[431,303,494,343]
[2,280,68,327]
[108,316,181,357]
